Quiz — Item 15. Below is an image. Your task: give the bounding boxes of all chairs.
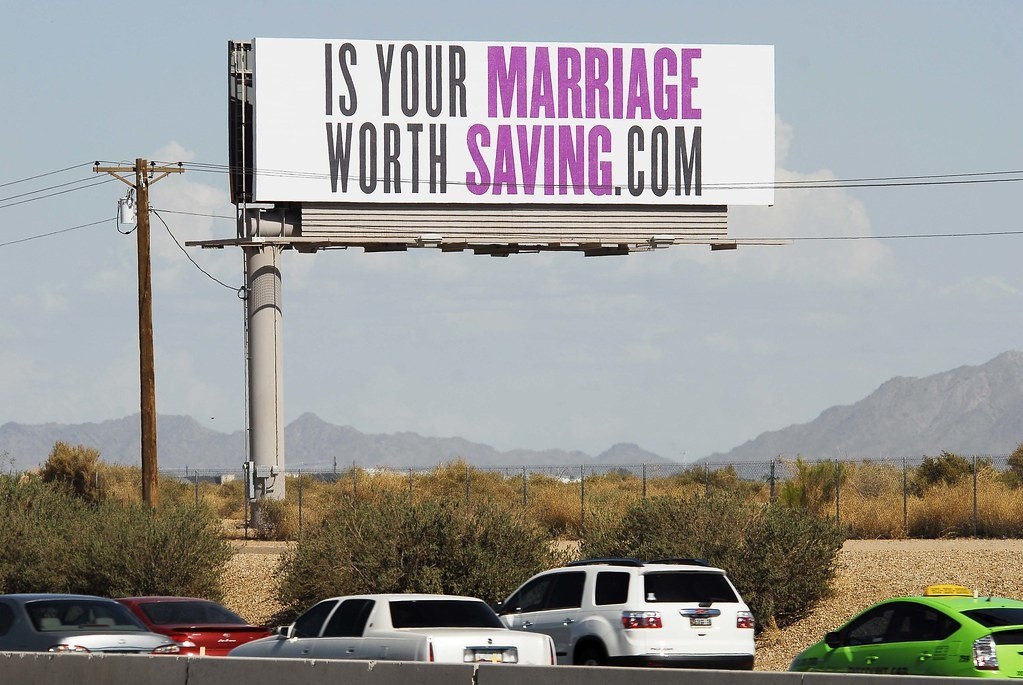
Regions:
[93,617,116,626]
[36,617,62,630]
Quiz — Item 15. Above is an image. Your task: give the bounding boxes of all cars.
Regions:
[229,594,558,667]
[789,585,1023,678]
[1,593,183,656]
[111,598,272,655]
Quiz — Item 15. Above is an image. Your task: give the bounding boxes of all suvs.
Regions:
[489,556,757,672]
